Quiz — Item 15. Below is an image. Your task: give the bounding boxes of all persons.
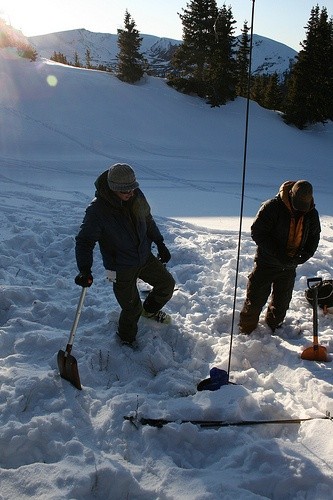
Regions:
[74,162,176,352]
[239,180,322,335]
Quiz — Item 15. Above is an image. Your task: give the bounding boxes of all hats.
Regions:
[108,163,139,190]
[291,181,312,212]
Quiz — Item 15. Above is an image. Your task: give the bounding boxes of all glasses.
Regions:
[119,189,131,194]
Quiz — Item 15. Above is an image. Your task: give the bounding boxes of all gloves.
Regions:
[75,271,94,287]
[156,244,171,264]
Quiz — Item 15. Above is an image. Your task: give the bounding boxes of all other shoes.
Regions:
[141,306,172,324]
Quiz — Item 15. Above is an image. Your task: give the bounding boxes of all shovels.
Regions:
[299,277,329,362]
[55,286,88,392]
